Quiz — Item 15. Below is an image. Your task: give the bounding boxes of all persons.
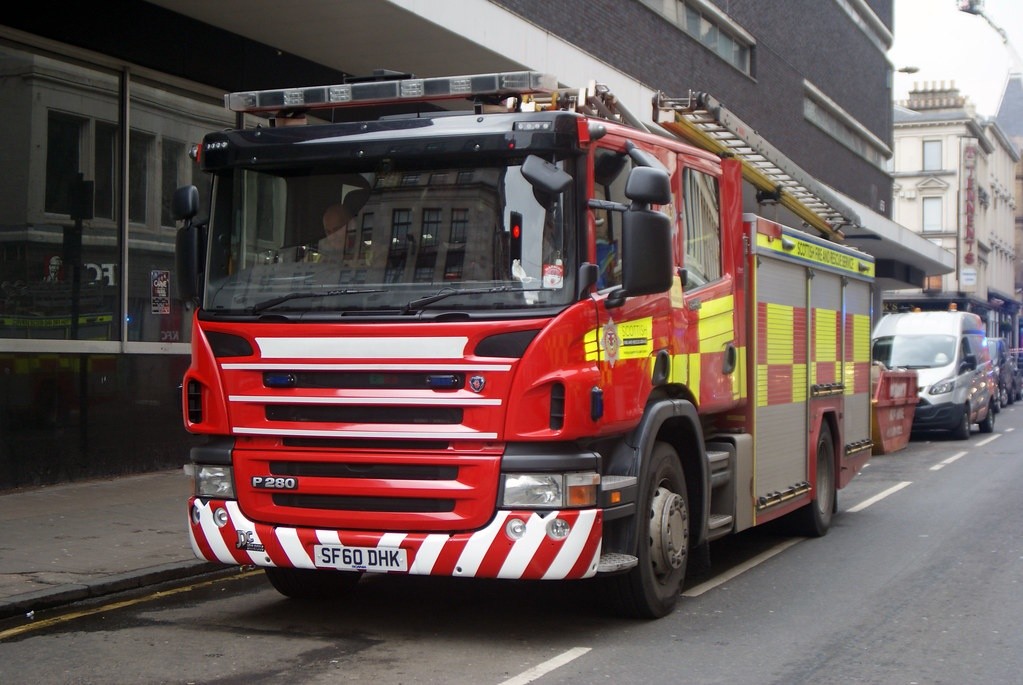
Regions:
[287,203,389,283]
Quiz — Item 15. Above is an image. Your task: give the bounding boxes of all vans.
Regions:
[871,303,999,438]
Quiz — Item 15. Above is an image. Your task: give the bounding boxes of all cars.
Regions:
[986,334,1023,407]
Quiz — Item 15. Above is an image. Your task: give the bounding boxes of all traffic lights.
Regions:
[44,167,84,218]
[510,208,524,262]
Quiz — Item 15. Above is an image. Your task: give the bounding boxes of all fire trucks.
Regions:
[172,71,875,620]
